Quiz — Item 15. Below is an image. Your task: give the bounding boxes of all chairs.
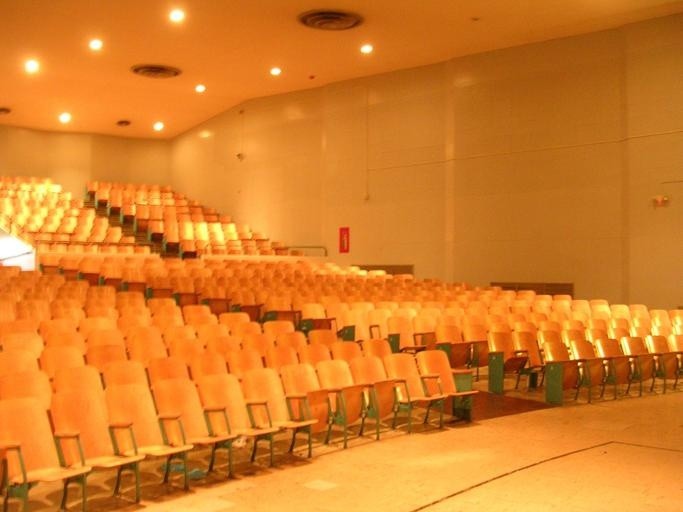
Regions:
[87,182,306,257]
[0,175,162,257]
